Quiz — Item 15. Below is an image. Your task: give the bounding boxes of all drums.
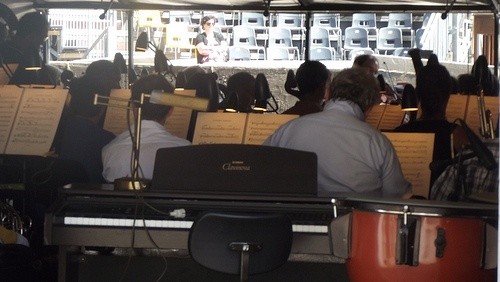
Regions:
[347,201,498,282]
[0,229,34,282]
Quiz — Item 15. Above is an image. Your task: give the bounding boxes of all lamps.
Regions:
[135,32,159,53]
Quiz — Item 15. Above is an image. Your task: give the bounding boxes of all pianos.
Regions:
[40,184,350,272]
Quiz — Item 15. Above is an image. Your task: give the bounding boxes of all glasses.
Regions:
[204,22,215,26]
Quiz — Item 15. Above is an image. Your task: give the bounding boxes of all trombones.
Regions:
[476,88,495,139]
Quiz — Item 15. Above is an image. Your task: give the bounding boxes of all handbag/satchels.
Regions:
[430,120,499,202]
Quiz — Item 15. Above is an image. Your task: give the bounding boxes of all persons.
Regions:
[101,74,192,181]
[351,54,381,80]
[54,74,118,185]
[86,59,123,97]
[217,71,264,113]
[194,15,229,64]
[0,11,50,66]
[260,67,414,201]
[183,64,207,90]
[391,63,466,183]
[281,61,333,116]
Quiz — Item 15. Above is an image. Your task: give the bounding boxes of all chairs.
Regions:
[169,11,195,33]
[344,26,370,61]
[309,46,335,61]
[351,13,378,38]
[163,23,196,60]
[187,211,293,282]
[310,26,331,48]
[268,27,300,60]
[202,10,229,35]
[388,12,415,48]
[277,13,306,35]
[241,13,269,32]
[228,46,251,61]
[374,27,406,57]
[135,9,164,42]
[313,14,343,49]
[232,25,267,60]
[264,46,291,60]
